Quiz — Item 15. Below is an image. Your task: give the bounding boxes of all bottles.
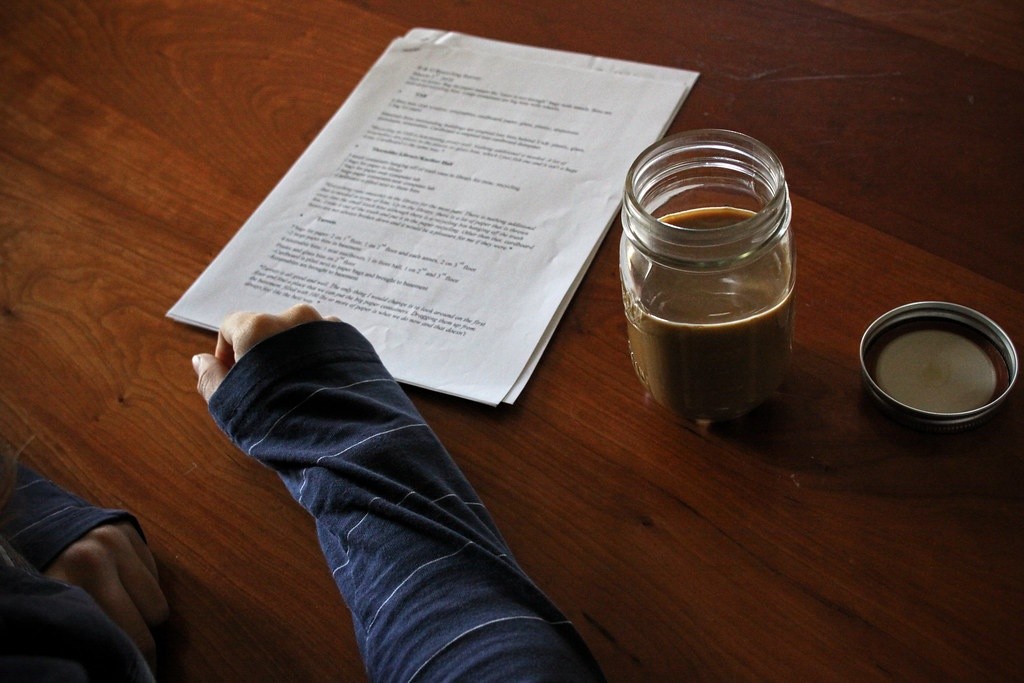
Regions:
[618,129,797,425]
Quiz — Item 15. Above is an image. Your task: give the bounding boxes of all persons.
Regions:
[0,302,606,683]
